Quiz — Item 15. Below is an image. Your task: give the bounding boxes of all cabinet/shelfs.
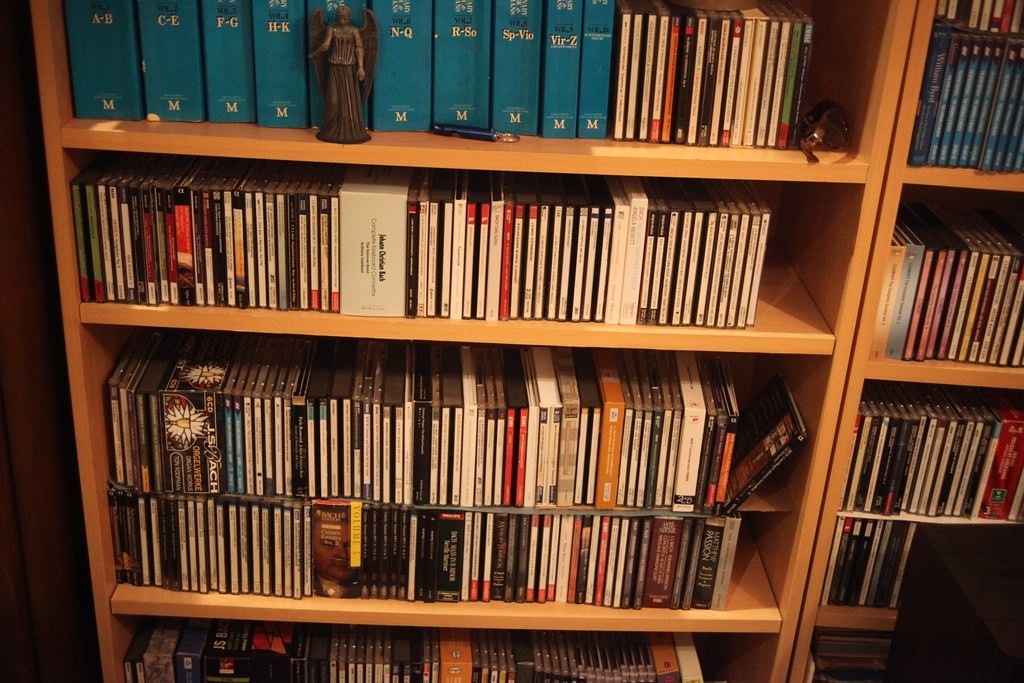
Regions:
[787,0,1024,681]
[33,0,920,681]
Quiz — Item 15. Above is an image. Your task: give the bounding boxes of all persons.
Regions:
[308,5,379,145]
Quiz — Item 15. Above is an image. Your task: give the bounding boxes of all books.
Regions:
[863,201,1023,372]
[819,515,918,611]
[69,156,772,334]
[65,0,816,148]
[839,391,1024,525]
[123,619,705,682]
[102,343,809,512]
[110,482,742,612]
[909,0,1023,174]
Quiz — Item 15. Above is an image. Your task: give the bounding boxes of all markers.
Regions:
[430,122,520,143]
